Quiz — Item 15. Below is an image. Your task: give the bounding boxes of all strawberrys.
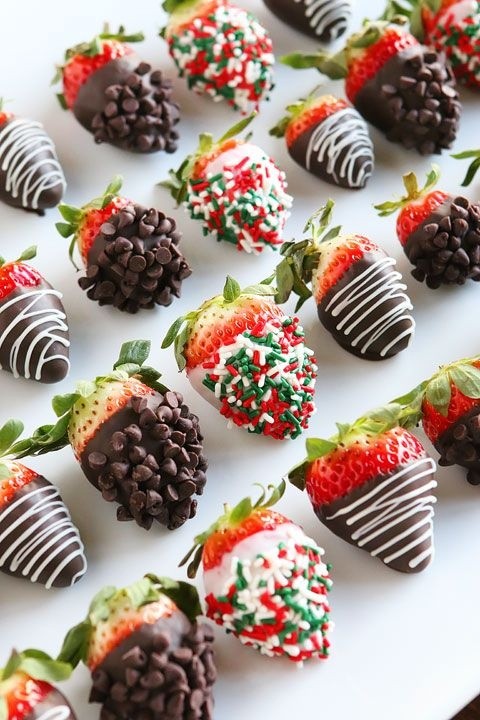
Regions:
[0,0,480,720]
